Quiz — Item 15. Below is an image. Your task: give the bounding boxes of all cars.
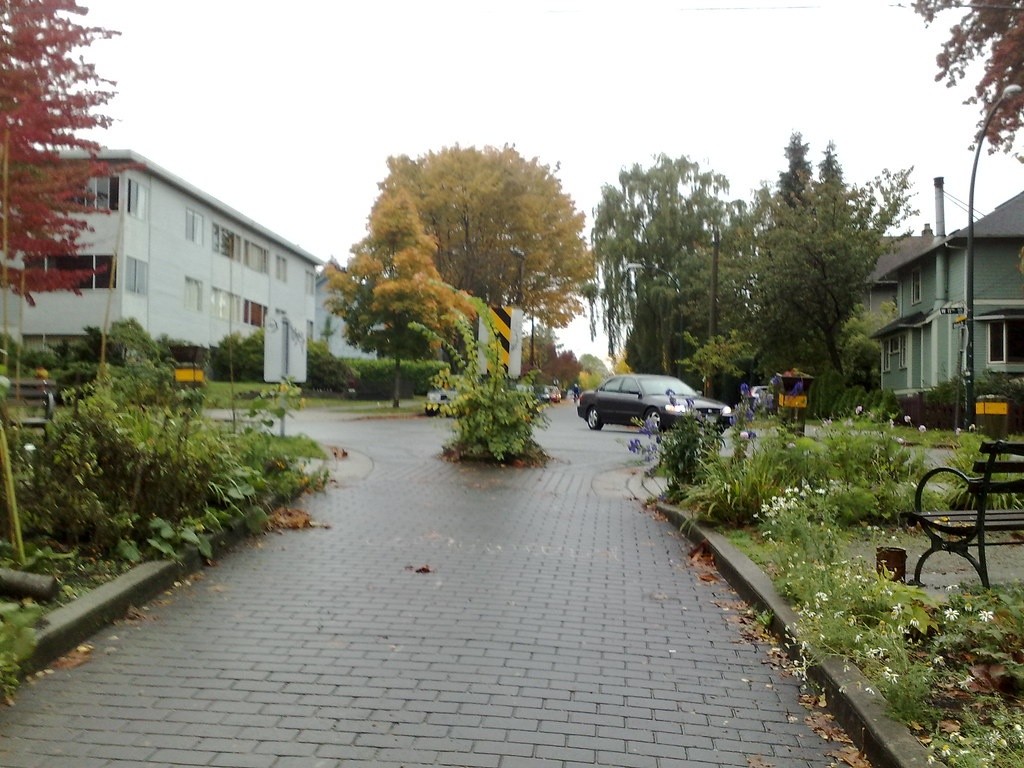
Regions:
[751,386,772,402]
[577,374,734,438]
[425,383,567,417]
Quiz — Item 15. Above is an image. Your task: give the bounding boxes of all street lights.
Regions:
[628,262,684,379]
[962,84,1024,432]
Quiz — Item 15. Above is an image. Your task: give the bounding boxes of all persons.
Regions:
[572,383,580,399]
[33,368,55,424]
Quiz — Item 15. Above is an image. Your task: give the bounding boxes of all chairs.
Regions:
[899,441,1024,590]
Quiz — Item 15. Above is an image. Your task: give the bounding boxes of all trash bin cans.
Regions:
[172,361,203,418]
[974,394,1011,442]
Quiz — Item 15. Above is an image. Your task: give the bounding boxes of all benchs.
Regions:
[8,378,58,433]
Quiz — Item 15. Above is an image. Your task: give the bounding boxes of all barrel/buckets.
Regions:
[876,547,906,583]
